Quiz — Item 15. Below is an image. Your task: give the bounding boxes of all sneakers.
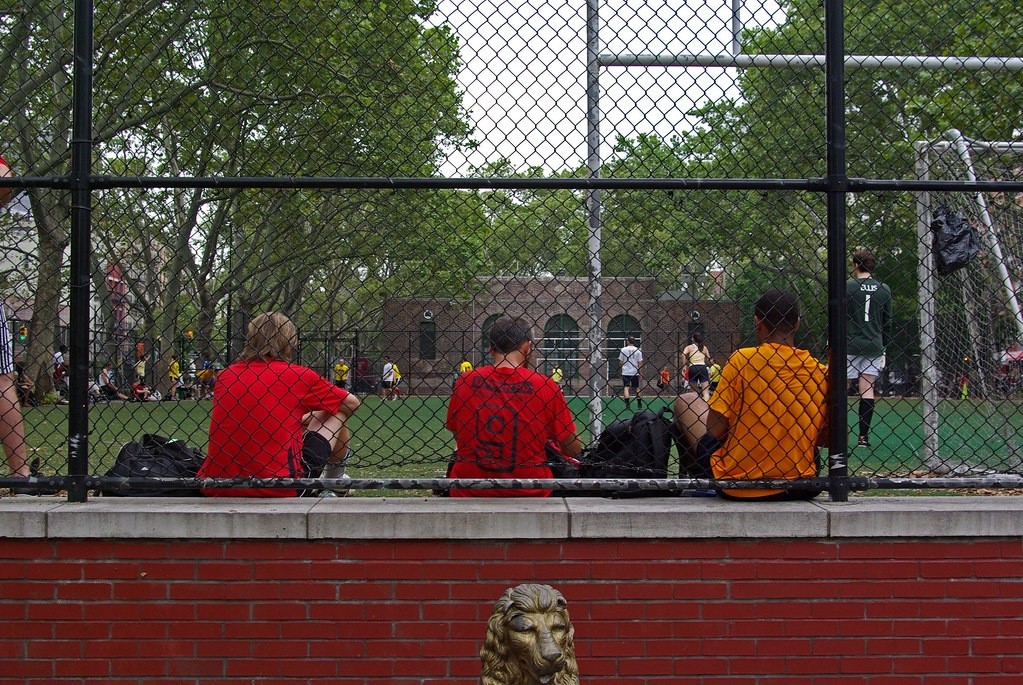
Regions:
[625,403,630,410]
[320,474,356,496]
[10,456,61,498]
[858,434,870,447]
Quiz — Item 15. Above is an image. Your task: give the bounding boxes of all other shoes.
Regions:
[637,404,643,409]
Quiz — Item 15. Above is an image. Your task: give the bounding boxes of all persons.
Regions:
[388,360,402,400]
[618,336,643,410]
[846,252,891,448]
[682,332,722,402]
[0,298,56,497]
[334,358,349,388]
[15,362,32,407]
[99,362,136,401]
[196,365,216,397]
[196,312,361,498]
[380,356,396,402]
[460,357,472,372]
[169,354,179,400]
[445,315,581,498]
[657,366,670,388]
[53,344,68,396]
[132,354,151,401]
[551,365,564,396]
[675,290,829,502]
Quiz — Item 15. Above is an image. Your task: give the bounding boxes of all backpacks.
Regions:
[584,406,680,501]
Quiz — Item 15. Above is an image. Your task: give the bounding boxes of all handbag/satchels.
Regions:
[93,432,206,497]
